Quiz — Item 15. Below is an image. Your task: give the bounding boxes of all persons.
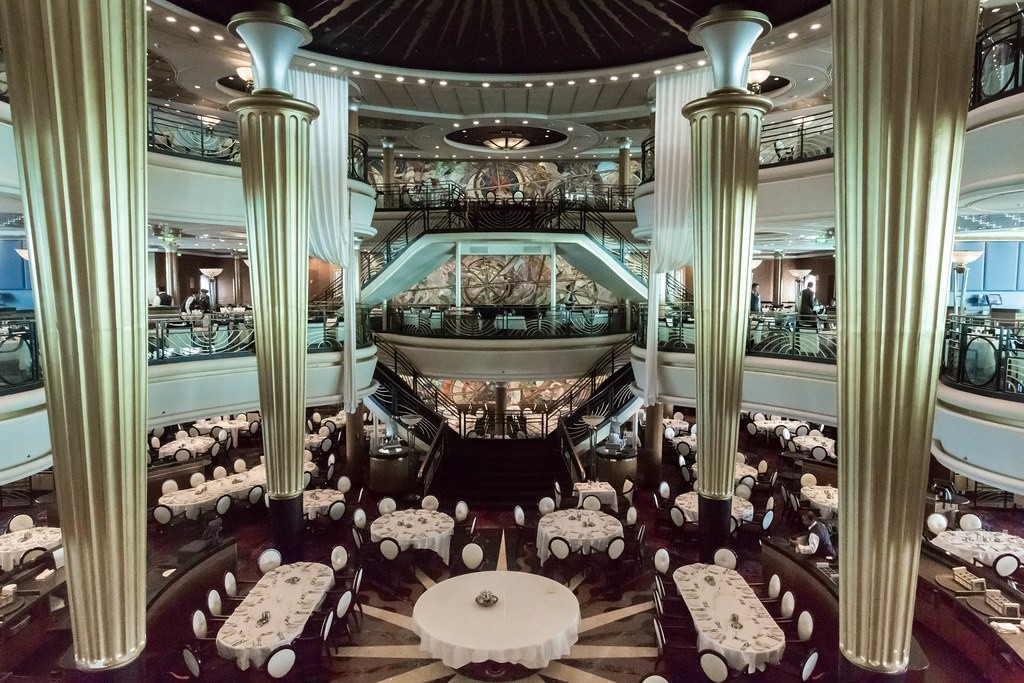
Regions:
[799,282,835,315]
[751,283,760,312]
[564,285,576,310]
[789,511,835,556]
[152,284,175,306]
[182,288,211,314]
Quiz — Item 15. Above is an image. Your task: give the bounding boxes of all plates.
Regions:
[475,595,498,607]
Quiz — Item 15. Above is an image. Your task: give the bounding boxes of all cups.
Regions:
[479,590,494,602]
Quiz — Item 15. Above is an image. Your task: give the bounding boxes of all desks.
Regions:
[1,1,1024,683]
